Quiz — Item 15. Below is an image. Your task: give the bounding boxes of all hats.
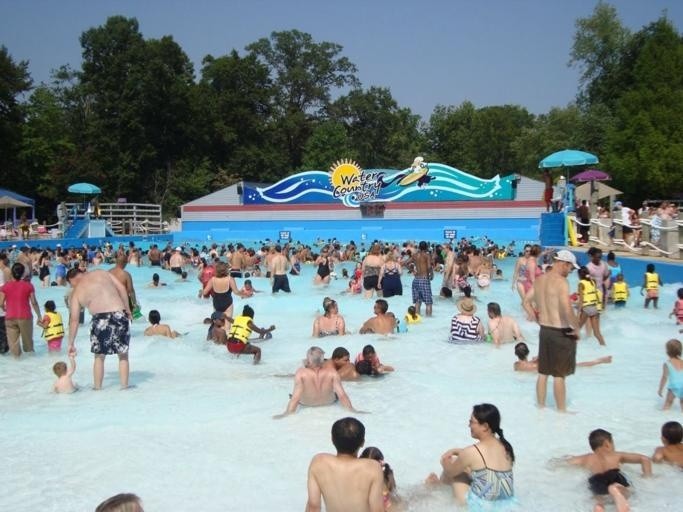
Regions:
[175,247,182,251]
[457,298,477,315]
[553,250,580,269]
[614,201,622,207]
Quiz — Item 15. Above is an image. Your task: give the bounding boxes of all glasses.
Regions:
[322,250,329,253]
[525,249,532,253]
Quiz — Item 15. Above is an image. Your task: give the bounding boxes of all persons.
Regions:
[511,244,682,413]
[424,404,517,512]
[95,492,143,512]
[538,168,554,212]
[1,240,185,395]
[648,420,683,474]
[546,428,653,512]
[359,446,397,509]
[185,236,522,420]
[577,199,678,249]
[4,202,66,240]
[304,417,409,512]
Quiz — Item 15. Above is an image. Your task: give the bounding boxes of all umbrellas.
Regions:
[575,182,623,201]
[576,170,612,187]
[67,182,100,211]
[538,150,599,184]
